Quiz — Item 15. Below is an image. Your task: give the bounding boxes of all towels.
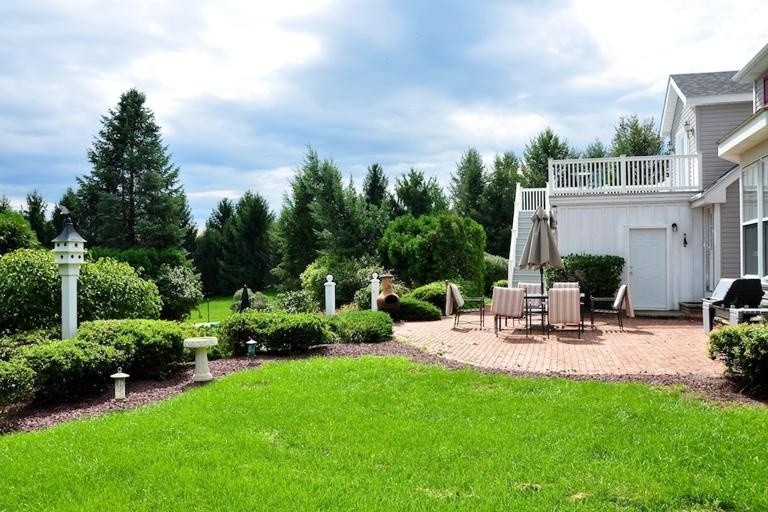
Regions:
[701,277,765,332]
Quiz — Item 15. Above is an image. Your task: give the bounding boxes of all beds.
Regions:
[518,206,566,294]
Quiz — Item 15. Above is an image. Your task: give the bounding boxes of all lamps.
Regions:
[450,285,484,330]
[590,285,627,332]
[552,282,583,326]
[518,283,544,325]
[546,288,584,339]
[493,287,531,338]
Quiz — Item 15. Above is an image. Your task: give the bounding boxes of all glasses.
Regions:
[667,141,674,152]
[684,121,693,139]
